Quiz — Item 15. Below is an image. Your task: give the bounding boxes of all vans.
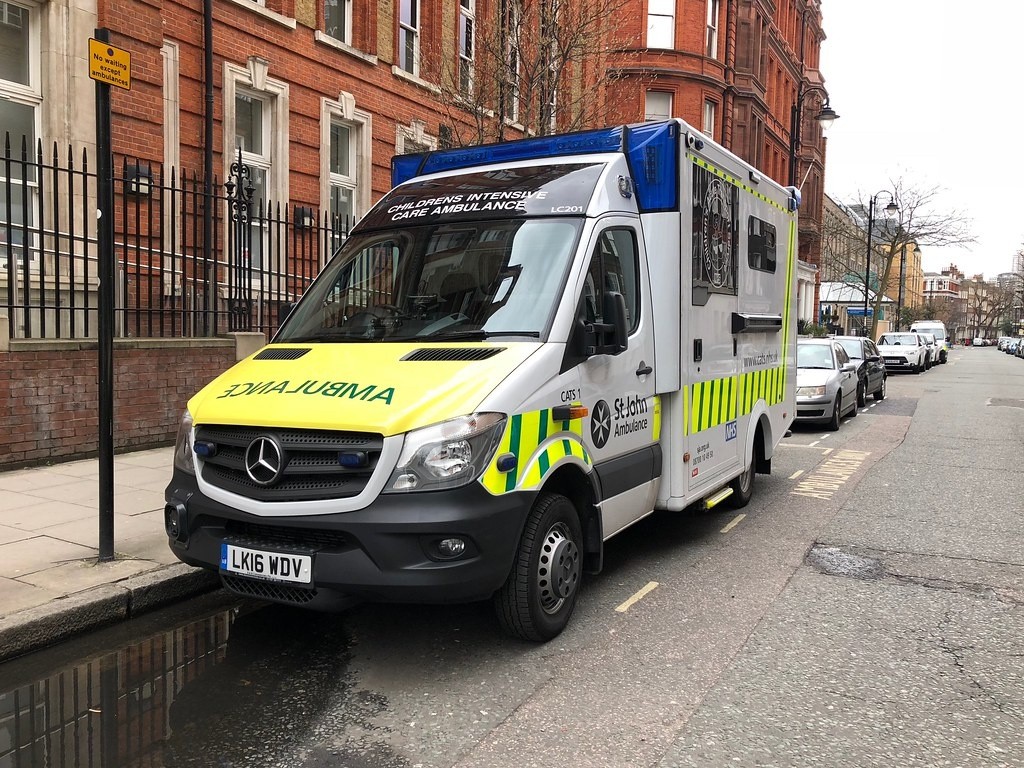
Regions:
[909,319,948,364]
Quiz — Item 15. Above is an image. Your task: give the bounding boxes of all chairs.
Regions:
[425,273,491,325]
[516,258,564,329]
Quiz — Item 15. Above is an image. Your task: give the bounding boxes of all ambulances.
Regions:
[164,117,816,645]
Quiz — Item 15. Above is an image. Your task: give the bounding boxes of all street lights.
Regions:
[787,9,841,189]
[861,189,900,337]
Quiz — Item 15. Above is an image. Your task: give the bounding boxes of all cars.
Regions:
[824,335,889,407]
[796,338,860,431]
[910,332,942,369]
[997,337,1024,359]
[876,332,927,376]
[973,337,998,347]
